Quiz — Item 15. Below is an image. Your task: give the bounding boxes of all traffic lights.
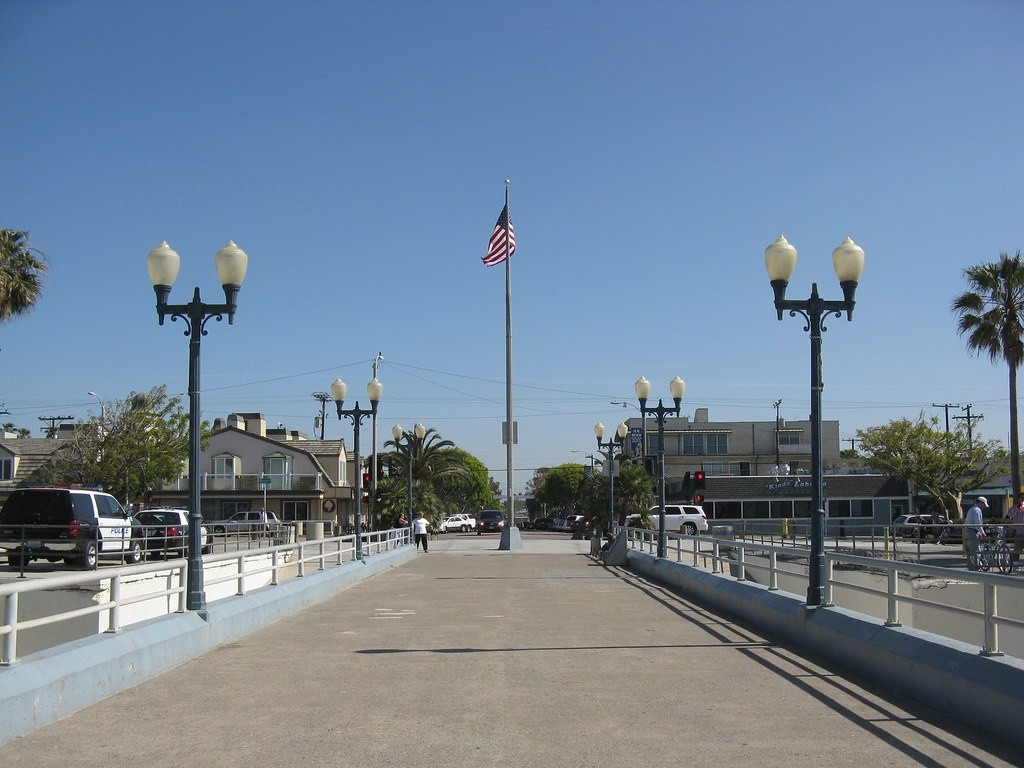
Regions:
[363,474,370,489]
[696,495,704,502]
[695,471,706,490]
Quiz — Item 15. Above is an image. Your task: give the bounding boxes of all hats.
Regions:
[978,496,989,508]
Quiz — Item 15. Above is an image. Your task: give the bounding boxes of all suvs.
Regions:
[0,487,146,570]
[201,510,282,537]
[624,505,708,536]
[892,514,954,537]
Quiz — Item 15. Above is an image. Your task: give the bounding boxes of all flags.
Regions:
[481,202,516,267]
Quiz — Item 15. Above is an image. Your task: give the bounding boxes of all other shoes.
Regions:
[426,550,428,553]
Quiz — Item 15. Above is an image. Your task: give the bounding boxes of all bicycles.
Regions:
[978,535,1013,576]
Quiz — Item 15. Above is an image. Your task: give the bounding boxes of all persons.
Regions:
[965,497,990,572]
[1003,492,1024,574]
[413,511,430,553]
[393,513,408,545]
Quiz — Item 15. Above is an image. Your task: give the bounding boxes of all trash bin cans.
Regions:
[680,525,687,534]
[712,525,734,551]
[591,537,602,554]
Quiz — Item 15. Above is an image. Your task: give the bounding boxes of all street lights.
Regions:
[571,449,591,458]
[392,423,427,542]
[594,421,629,534]
[764,233,867,610]
[88,391,105,458]
[146,239,249,612]
[634,375,686,555]
[330,377,383,560]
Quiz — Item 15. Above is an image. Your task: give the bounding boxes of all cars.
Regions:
[434,509,585,536]
[133,508,214,558]
